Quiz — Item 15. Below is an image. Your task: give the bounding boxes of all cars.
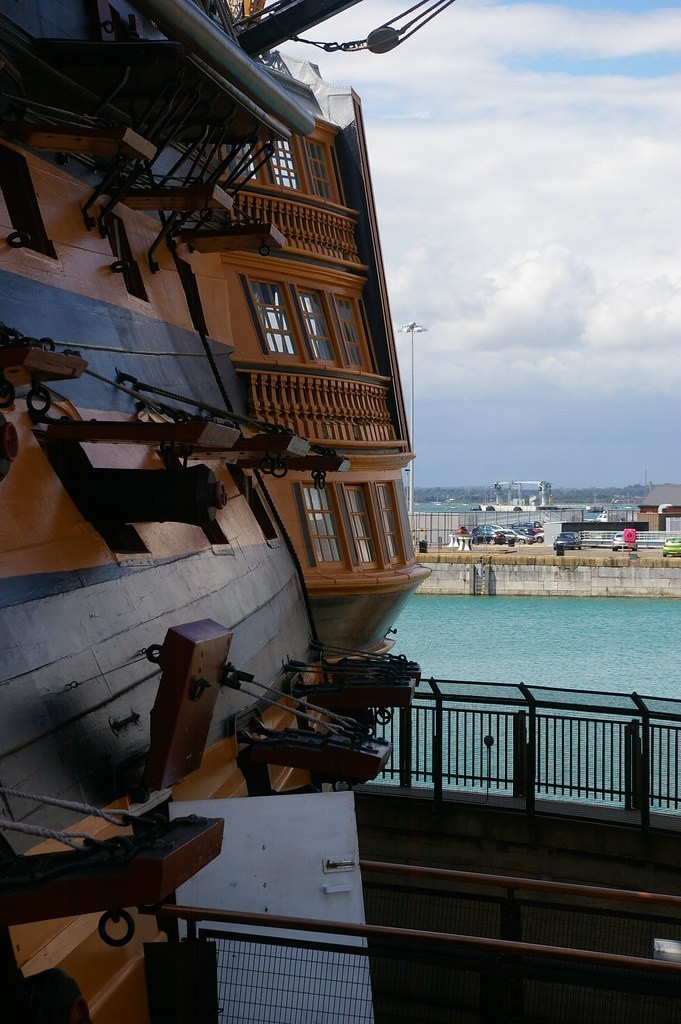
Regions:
[663,538,681,557]
[471,522,544,546]
[553,533,583,549]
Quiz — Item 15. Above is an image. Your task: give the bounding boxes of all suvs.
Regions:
[612,534,637,552]
